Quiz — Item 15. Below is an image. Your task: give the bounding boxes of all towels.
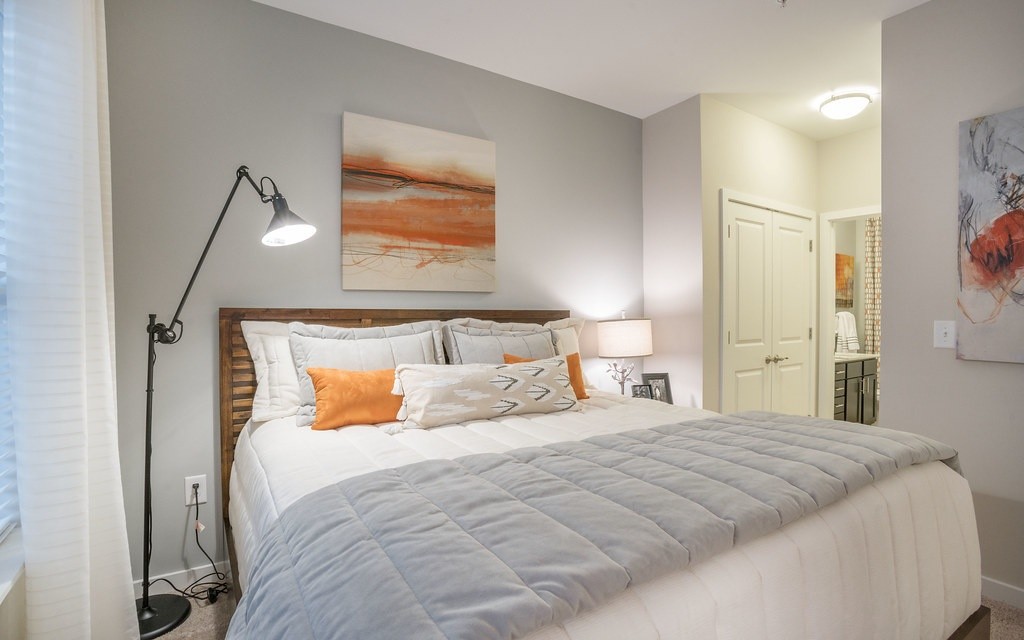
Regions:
[836,311,860,354]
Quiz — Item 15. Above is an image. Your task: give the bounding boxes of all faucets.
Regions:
[835,331,838,352]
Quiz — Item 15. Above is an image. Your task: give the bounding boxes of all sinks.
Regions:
[835,356,850,361]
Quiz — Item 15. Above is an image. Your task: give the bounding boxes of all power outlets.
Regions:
[932,320,957,350]
[184,473,207,507]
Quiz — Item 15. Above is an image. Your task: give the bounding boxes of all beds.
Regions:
[216,305,990,640]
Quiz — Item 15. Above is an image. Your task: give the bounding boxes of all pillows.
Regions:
[389,352,585,430]
[502,351,590,400]
[443,317,586,359]
[304,366,405,429]
[238,318,301,423]
[287,320,447,427]
[440,324,560,366]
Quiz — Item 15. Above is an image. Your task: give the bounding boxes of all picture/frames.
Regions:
[642,371,673,405]
[631,384,654,399]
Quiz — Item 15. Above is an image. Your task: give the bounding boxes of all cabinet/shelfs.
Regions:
[835,359,879,425]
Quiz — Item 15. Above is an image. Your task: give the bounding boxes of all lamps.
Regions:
[135,162,318,639]
[820,92,871,122]
[594,309,652,395]
